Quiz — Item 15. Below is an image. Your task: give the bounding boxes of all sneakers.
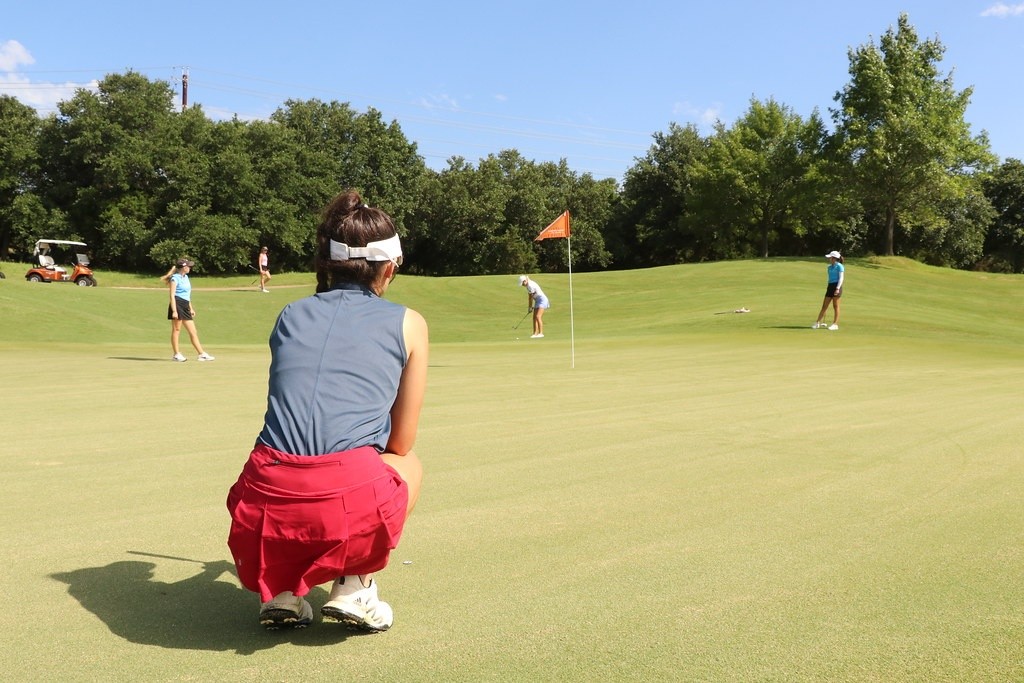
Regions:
[198,352,214,362]
[321,574,393,630]
[173,354,187,362]
[259,589,313,629]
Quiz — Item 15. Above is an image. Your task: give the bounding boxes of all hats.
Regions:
[329,230,403,268]
[262,246,268,251]
[825,250,841,259]
[519,275,526,286]
[176,259,194,267]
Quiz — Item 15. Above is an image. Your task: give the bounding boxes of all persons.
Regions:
[812,251,844,330]
[518,276,549,338]
[258,247,271,293]
[164,259,215,361]
[226,191,428,630]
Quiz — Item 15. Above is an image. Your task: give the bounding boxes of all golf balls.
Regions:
[516,337,519,339]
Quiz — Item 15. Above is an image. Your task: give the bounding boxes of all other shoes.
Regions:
[812,323,819,329]
[261,289,269,293]
[828,324,838,330]
[530,332,544,339]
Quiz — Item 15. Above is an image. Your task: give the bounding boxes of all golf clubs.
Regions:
[821,312,828,326]
[512,307,534,329]
[248,265,260,272]
